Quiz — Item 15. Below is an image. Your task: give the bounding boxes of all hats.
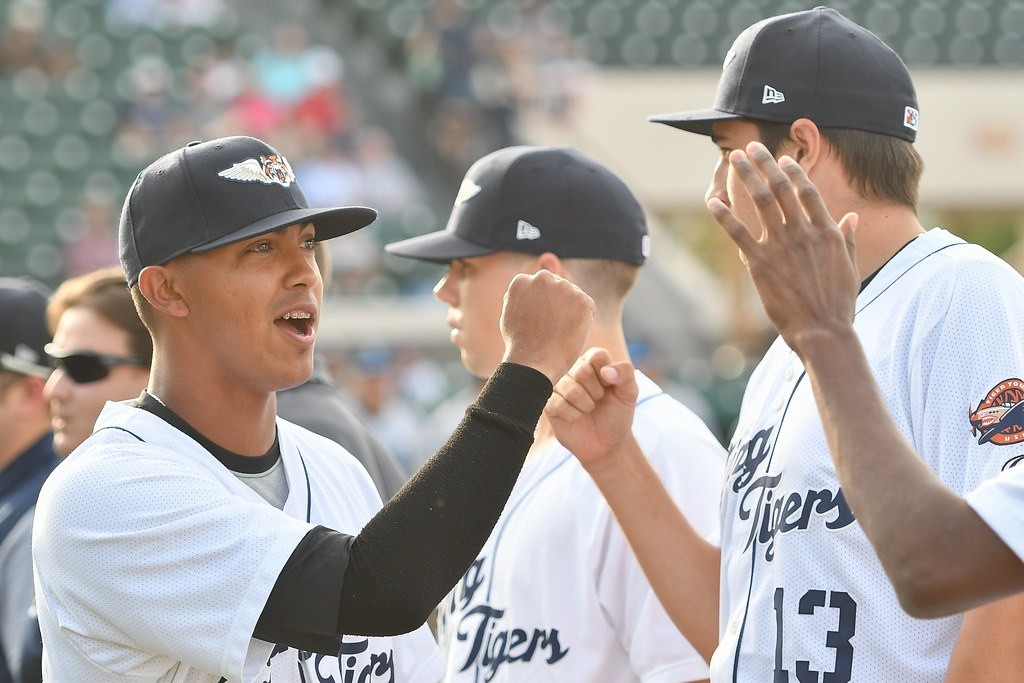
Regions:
[0,275,63,380]
[116,135,378,286]
[649,4,920,142]
[382,145,649,264]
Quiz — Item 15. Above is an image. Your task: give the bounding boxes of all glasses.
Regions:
[39,341,149,384]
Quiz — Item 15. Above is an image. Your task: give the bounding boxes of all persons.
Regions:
[29,132,593,683]
[547,6,1024,683]
[708,141,1024,620]
[0,263,442,683]
[384,147,728,683]
